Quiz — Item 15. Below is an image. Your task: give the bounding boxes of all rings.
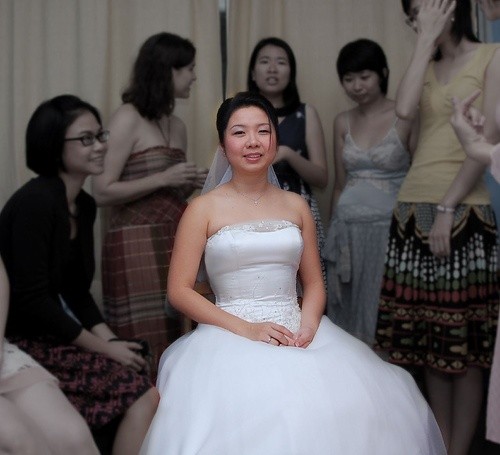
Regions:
[267,337,271,343]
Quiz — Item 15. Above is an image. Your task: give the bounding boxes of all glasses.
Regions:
[63,129,110,147]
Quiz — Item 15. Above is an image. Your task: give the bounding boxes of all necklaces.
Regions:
[232,181,268,206]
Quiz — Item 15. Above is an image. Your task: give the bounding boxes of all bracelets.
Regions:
[435,204,455,214]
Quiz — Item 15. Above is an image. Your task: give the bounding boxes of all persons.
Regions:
[246,36,328,314]
[139,91,448,455]
[93,31,210,385]
[0,258,101,455]
[321,37,420,361]
[395,1,499,455]
[0,94,160,455]
